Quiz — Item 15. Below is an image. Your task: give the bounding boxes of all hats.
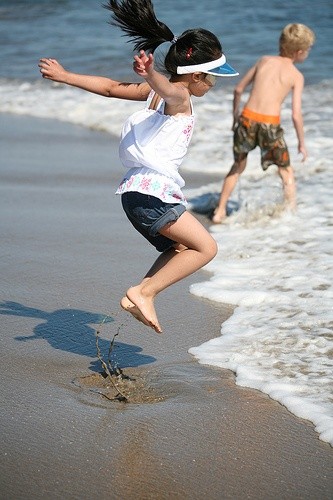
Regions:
[177,53,240,77]
